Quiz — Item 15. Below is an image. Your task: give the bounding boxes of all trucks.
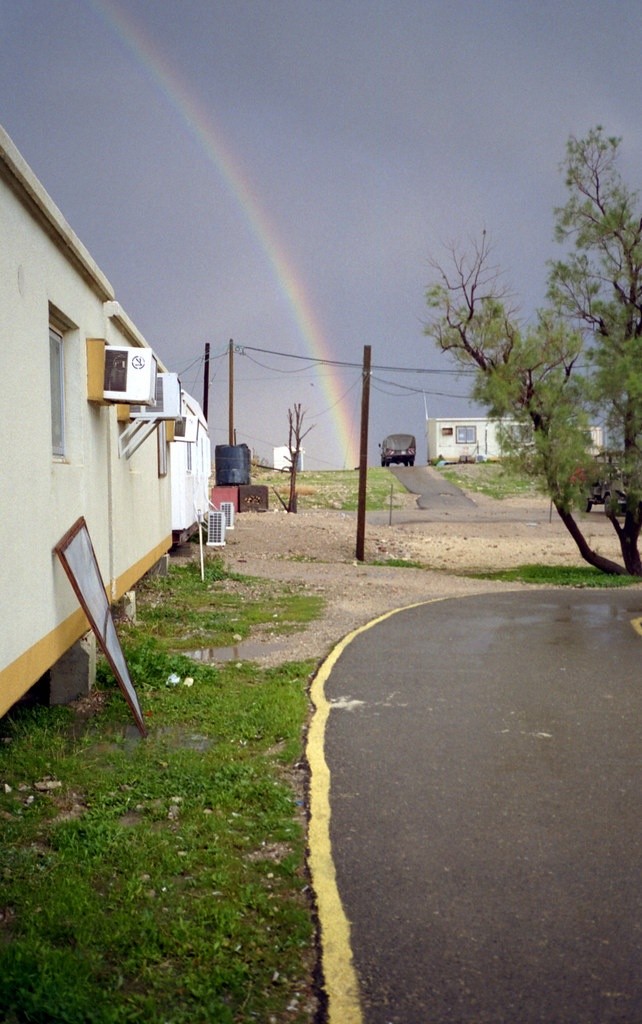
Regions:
[377,434,418,466]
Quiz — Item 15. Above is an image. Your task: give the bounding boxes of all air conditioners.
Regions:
[206,510,227,546]
[173,415,200,443]
[129,372,183,421]
[104,345,158,406]
[475,454,487,463]
[219,502,234,530]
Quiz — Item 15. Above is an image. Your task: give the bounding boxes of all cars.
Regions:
[577,447,628,513]
